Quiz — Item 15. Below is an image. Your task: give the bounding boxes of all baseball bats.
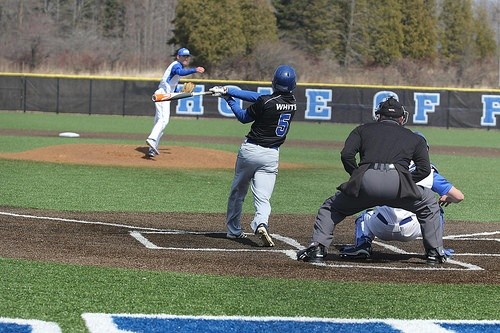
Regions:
[152,91,213,102]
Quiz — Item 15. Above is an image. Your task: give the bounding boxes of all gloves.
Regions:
[209,86,226,96]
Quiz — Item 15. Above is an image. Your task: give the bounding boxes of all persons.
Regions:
[296,96,450,264]
[146,47,205,158]
[208,65,297,248]
[340,130,466,259]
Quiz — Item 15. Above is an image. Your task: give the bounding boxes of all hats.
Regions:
[375,97,404,117]
[177,47,191,56]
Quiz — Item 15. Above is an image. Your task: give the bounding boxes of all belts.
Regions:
[368,163,396,170]
[377,213,412,225]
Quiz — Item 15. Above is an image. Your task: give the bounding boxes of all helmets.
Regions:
[414,132,430,150]
[272,65,296,92]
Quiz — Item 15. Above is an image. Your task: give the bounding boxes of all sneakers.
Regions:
[226,232,248,239]
[426,255,447,265]
[148,147,155,156]
[146,139,160,155]
[257,224,275,247]
[339,234,373,259]
[297,244,327,261]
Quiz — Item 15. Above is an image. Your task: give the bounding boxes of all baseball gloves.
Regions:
[181,82,195,93]
